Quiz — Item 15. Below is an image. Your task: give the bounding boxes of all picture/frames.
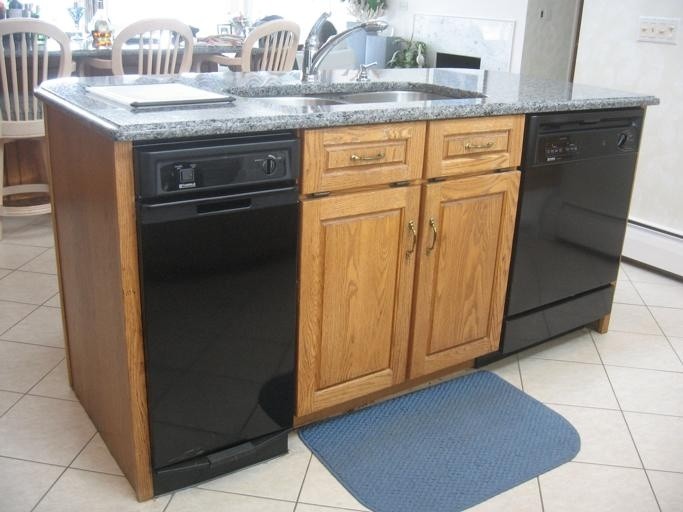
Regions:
[217,24,232,35]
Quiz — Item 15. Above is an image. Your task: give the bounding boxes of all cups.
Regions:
[217,25,232,36]
[246,27,255,34]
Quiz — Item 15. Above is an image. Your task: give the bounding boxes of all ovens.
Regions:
[132,131,300,496]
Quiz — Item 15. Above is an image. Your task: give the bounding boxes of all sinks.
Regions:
[329,90,457,103]
[244,96,349,105]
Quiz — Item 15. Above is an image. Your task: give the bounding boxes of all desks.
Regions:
[0,39,303,196]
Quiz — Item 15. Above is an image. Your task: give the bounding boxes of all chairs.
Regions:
[194,19,301,73]
[1,19,73,218]
[77,18,194,76]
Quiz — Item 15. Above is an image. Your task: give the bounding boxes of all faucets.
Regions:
[302,11,388,83]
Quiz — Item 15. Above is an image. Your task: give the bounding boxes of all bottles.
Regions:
[0,0,46,49]
[89,0,113,41]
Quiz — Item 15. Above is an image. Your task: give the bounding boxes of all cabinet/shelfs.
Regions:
[293,115,526,438]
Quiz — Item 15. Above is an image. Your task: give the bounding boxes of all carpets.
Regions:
[298,369,581,512]
